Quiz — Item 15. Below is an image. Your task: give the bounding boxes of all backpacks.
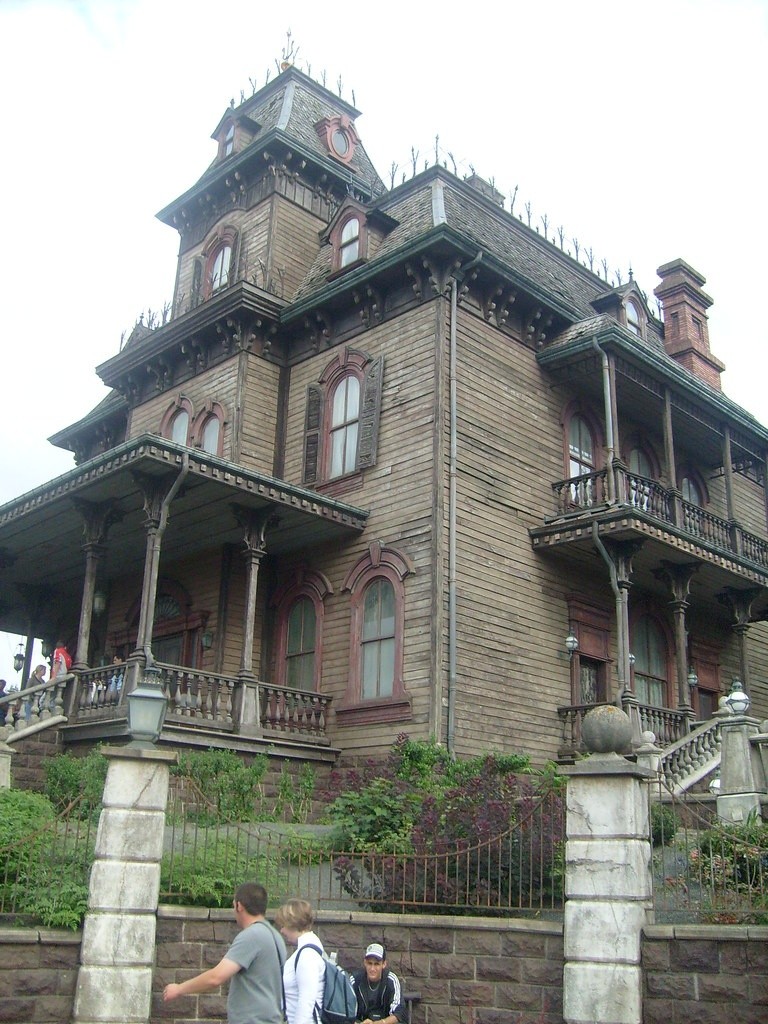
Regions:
[294,944,360,1023]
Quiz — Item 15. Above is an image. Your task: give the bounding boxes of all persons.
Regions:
[0,639,126,727]
[162,882,406,1024]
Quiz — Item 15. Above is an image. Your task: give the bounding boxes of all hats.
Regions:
[363,943,386,961]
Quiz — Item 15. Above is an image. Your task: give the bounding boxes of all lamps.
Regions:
[13,618,26,673]
[726,688,752,715]
[41,635,54,660]
[564,626,578,660]
[629,652,637,667]
[125,661,170,750]
[202,629,212,648]
[687,665,698,693]
[708,767,721,795]
[731,679,742,691]
[99,655,110,667]
[94,583,106,619]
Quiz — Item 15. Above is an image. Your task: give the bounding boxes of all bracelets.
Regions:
[381,1019,385,1024]
